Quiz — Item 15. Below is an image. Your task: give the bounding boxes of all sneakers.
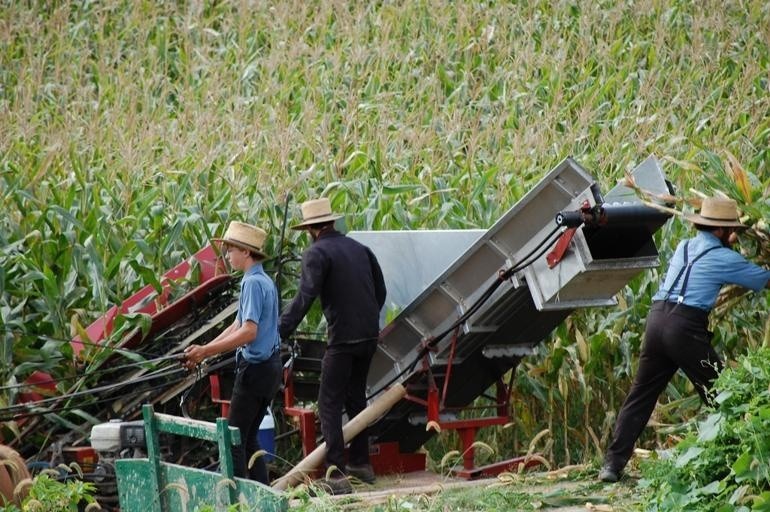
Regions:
[599,463,619,481]
[344,465,374,482]
[309,477,352,496]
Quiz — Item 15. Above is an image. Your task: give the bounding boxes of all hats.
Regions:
[684,197,748,229]
[210,221,273,263]
[290,197,345,229]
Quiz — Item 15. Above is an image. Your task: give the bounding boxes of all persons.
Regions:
[271,195,388,498]
[179,220,284,488]
[597,190,769,484]
